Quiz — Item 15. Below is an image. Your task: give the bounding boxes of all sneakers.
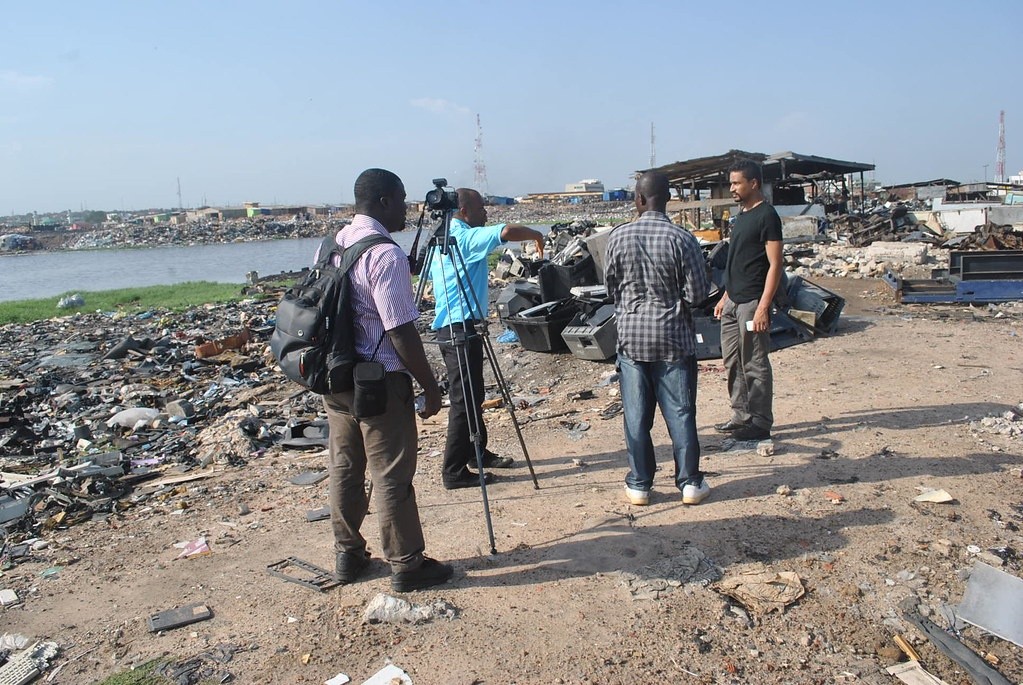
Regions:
[470,448,515,469]
[442,470,497,491]
[392,556,455,592]
[682,479,711,504]
[625,486,650,505]
[335,550,371,580]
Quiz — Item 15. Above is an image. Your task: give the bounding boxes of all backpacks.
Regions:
[269,226,403,394]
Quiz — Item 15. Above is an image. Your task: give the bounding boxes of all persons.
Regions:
[715,162,784,442]
[323,167,465,597]
[603,169,712,505]
[420,188,547,489]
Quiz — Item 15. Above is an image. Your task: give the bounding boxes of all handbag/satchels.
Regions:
[352,362,390,420]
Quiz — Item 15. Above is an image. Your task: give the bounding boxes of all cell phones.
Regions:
[746,320,753,331]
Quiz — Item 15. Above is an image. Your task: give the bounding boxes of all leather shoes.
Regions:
[713,419,746,432]
[730,425,771,441]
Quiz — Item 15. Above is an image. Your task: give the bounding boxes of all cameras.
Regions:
[426,178,460,211]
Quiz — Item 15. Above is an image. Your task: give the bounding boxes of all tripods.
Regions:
[363,215,539,554]
